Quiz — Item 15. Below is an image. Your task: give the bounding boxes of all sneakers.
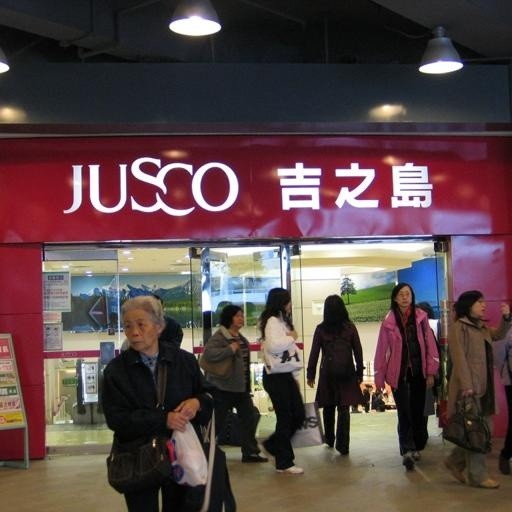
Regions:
[443,458,466,483]
[241,454,267,462]
[402,449,421,474]
[476,480,501,489]
[276,465,305,474]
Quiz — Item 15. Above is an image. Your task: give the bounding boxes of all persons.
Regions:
[372,281,440,470]
[154,294,184,347]
[102,296,237,512]
[198,305,269,463]
[306,294,363,455]
[499,330,511,475]
[444,290,511,489]
[257,287,304,474]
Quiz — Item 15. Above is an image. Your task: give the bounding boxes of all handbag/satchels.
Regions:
[261,339,305,375]
[105,435,170,494]
[198,331,230,378]
[442,391,493,454]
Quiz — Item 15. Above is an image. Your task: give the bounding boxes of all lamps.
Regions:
[385,24,464,75]
[0,47,11,74]
[169,0,223,37]
